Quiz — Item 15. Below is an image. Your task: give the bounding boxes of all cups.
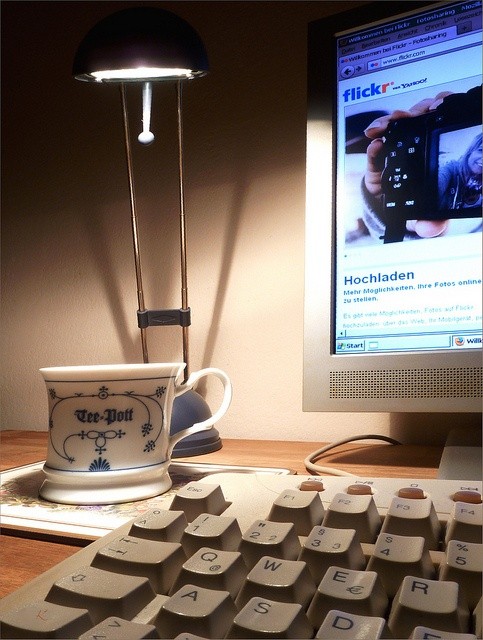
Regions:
[38,360,232,505]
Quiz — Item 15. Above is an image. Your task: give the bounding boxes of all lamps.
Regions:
[62,1,222,457]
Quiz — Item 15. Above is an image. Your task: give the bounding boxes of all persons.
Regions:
[439,133,482,207]
[346,92,448,240]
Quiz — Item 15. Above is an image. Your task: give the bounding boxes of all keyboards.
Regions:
[0,472,483,639]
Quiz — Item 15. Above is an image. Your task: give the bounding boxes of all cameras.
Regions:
[380,85,483,221]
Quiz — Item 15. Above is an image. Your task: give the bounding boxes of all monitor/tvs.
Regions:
[300,0,483,481]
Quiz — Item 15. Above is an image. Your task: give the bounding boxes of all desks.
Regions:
[0,429,483,640]
